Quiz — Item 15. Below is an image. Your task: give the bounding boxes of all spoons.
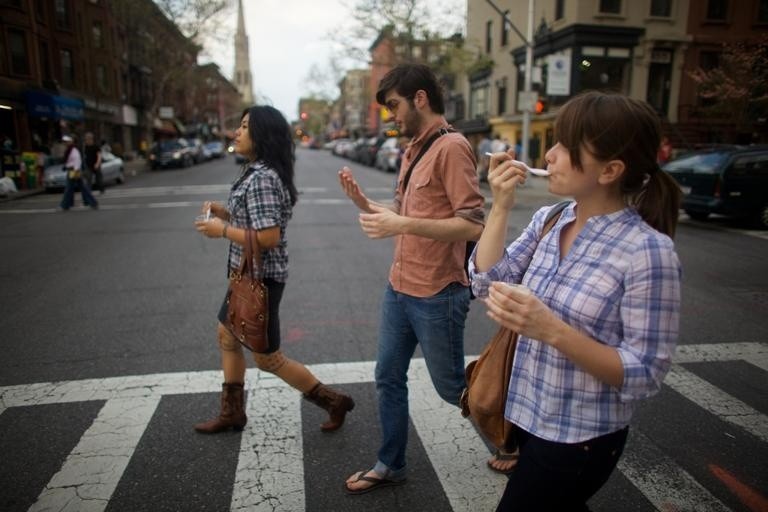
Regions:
[486,151,552,177]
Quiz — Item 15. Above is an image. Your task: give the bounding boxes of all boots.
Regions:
[194,383,247,435]
[302,381,355,432]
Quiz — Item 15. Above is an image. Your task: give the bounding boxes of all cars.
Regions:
[41,149,124,188]
[150,137,235,167]
[334,136,401,172]
[660,143,768,226]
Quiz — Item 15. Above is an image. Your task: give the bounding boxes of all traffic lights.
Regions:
[533,96,547,114]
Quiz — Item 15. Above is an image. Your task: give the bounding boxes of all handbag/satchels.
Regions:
[464,238,479,300]
[460,324,519,450]
[216,228,271,354]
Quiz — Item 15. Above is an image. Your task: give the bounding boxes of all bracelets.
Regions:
[223,222,228,239]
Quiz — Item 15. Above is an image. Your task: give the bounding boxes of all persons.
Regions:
[657,135,672,164]
[335,62,521,494]
[468,89,683,511]
[193,105,355,432]
[60,133,101,211]
[474,136,521,171]
[82,131,104,206]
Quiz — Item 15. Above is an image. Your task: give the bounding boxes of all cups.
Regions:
[194,211,215,221]
[487,280,530,300]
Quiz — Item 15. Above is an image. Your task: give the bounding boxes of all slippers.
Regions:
[341,467,401,495]
[486,450,519,473]
